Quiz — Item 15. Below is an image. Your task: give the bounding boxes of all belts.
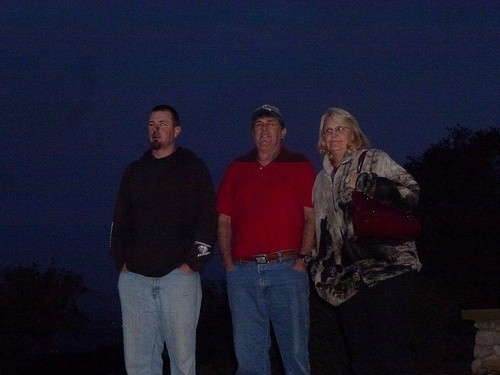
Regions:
[242,250,296,264]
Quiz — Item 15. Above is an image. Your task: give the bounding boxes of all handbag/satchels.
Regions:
[352,149,422,240]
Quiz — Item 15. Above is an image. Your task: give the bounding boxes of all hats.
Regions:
[253,104,285,124]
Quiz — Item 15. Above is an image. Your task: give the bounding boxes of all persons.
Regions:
[215,105,316,375]
[111,105,217,375]
[313,108,422,375]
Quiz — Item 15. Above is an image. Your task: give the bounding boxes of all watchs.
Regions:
[298,248,314,269]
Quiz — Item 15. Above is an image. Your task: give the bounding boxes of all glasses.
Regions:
[322,126,350,134]
